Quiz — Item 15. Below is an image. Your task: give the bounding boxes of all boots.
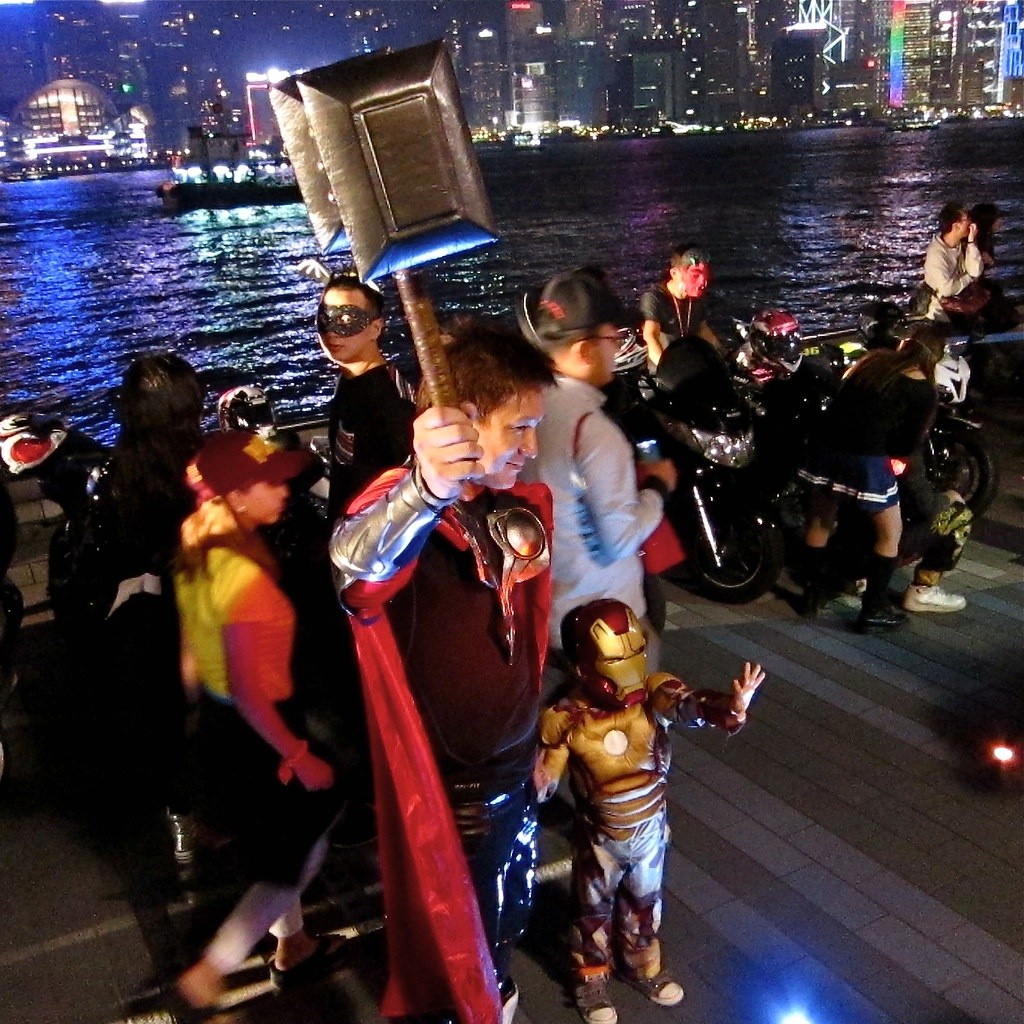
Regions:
[166,808,233,864]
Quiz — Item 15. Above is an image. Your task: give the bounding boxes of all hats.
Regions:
[972,203,1011,220]
[185,431,308,499]
[537,269,646,332]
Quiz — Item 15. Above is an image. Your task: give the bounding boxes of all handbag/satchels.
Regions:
[940,283,990,315]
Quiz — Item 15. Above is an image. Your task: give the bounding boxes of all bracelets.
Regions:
[278,740,307,784]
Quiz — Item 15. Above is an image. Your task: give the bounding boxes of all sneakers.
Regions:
[902,581,966,613]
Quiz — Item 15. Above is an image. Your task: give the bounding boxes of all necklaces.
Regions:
[674,295,691,335]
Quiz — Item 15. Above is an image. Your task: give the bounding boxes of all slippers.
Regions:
[269,935,347,985]
[164,987,246,1024]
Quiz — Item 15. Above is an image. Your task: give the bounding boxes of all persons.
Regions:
[315,278,416,523]
[331,318,552,1024]
[533,599,765,1024]
[166,431,352,1024]
[641,245,971,632]
[925,202,1001,295]
[519,267,677,707]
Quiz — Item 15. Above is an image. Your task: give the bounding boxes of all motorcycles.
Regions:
[605,341,788,603]
[737,307,1001,520]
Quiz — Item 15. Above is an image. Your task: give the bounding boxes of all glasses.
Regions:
[956,216,972,224]
[575,327,637,357]
[317,303,382,337]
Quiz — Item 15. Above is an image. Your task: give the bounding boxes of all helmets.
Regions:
[749,307,804,372]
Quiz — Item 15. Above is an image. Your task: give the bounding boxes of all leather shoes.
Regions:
[801,587,820,618]
[857,607,908,628]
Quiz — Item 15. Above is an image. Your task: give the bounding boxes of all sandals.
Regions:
[620,966,684,1005]
[575,982,617,1024]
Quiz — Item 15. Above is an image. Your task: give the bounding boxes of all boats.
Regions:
[153,171,305,221]
